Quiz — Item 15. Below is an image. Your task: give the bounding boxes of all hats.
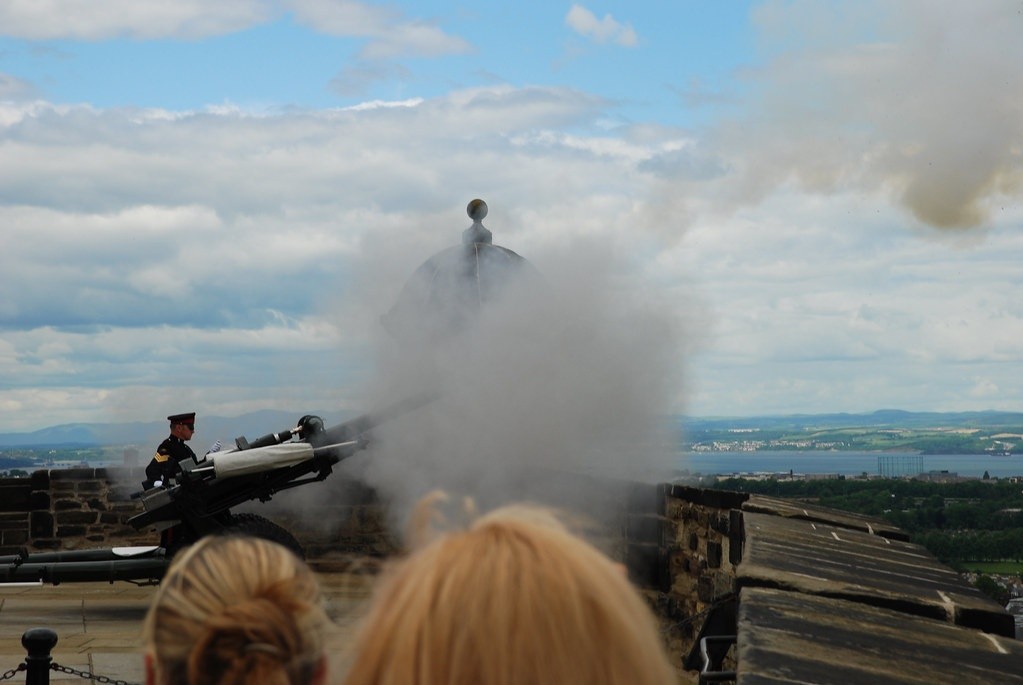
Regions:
[167,412,196,431]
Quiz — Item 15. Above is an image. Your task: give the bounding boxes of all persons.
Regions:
[145,534,329,685]
[345,505,681,685]
[145,412,220,486]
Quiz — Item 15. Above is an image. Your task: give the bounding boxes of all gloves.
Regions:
[209,440,221,453]
